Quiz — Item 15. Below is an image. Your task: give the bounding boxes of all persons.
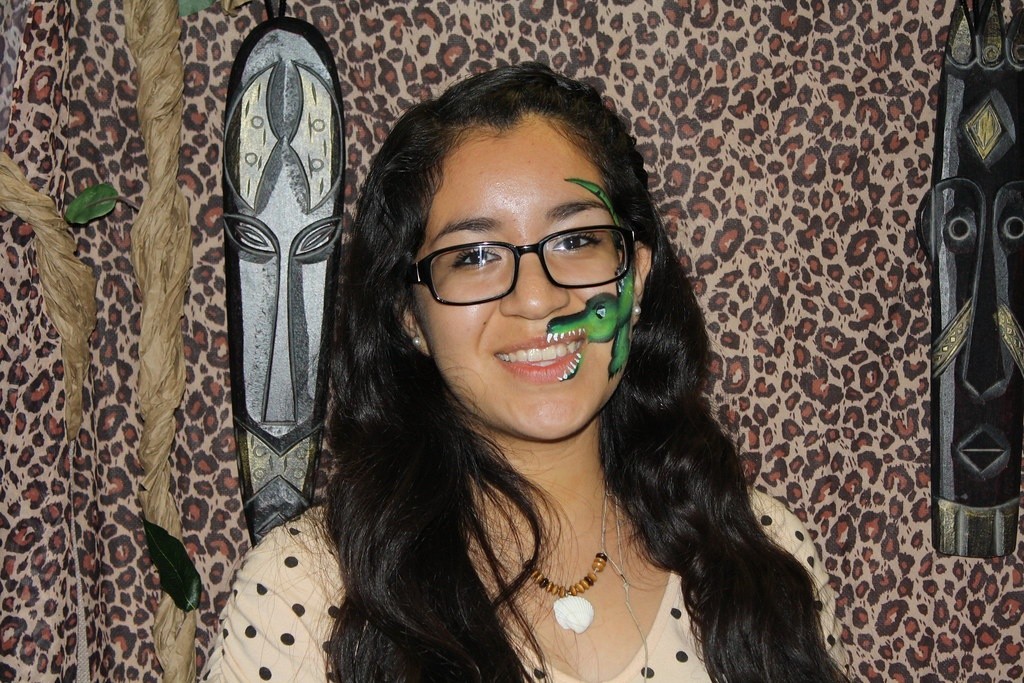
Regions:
[200,62,848,683]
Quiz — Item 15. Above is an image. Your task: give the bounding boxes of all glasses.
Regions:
[411,224,636,306]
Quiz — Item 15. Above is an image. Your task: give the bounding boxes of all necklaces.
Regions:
[478,463,648,683]
